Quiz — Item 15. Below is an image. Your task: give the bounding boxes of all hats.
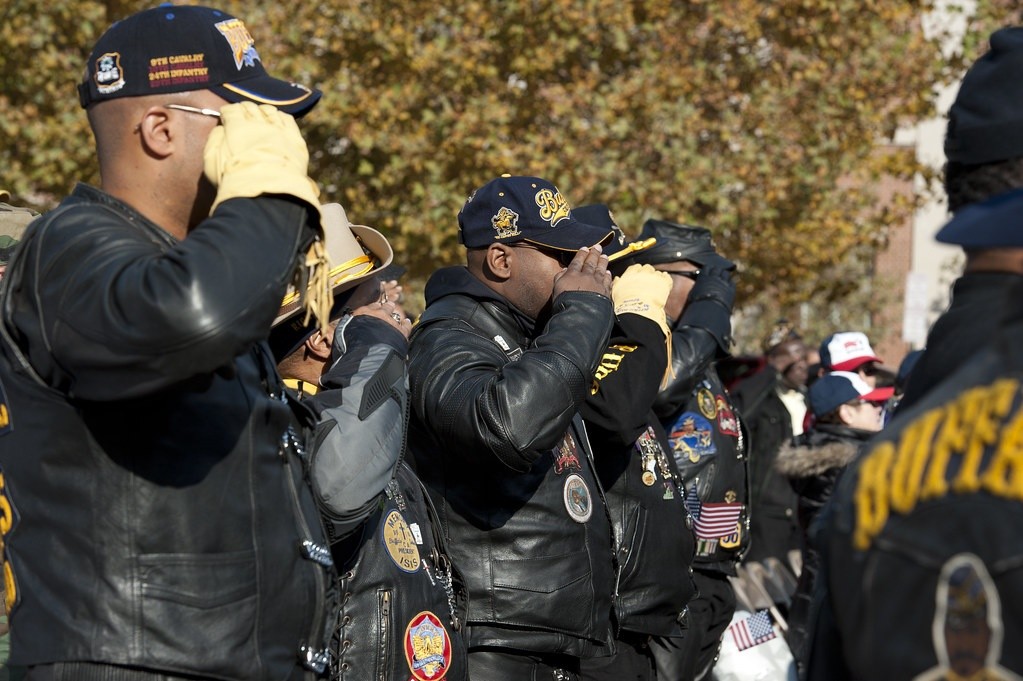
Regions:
[607,220,737,274]
[819,331,883,372]
[457,174,615,252]
[569,205,657,261]
[78,3,323,120]
[932,27,1023,247]
[894,348,927,395]
[269,202,394,328]
[803,372,895,417]
[267,260,406,363]
[760,321,807,375]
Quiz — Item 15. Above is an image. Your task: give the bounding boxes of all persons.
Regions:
[818,332,883,388]
[809,24,1023,681]
[781,371,893,641]
[0,0,749,681]
[729,320,810,577]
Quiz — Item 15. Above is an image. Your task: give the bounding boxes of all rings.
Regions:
[390,313,400,323]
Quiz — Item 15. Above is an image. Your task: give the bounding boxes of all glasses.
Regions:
[851,366,878,376]
[504,241,577,268]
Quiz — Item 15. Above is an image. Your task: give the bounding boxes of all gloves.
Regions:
[685,261,738,316]
[610,263,673,338]
[202,100,325,241]
